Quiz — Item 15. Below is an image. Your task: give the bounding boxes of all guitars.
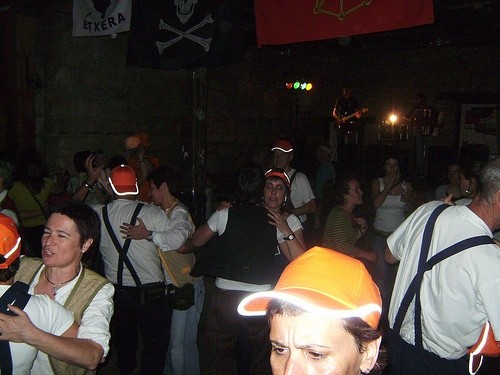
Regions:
[334,106,370,128]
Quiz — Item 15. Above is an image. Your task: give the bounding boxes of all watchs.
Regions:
[283,234,295,240]
[147,231,153,241]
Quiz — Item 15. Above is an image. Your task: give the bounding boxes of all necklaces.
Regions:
[44,268,80,295]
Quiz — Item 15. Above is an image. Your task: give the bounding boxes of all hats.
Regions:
[264,167,290,187]
[109,164,139,197]
[237,244,383,329]
[0,213,22,271]
[271,138,293,153]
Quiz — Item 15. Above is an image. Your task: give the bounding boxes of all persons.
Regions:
[0,158,115,375]
[92,150,110,176]
[7,148,54,256]
[385,156,500,375]
[109,155,128,171]
[333,84,360,145]
[124,133,155,177]
[313,142,490,350]
[95,165,174,375]
[404,95,443,137]
[236,246,388,375]
[191,140,317,375]
[67,151,107,204]
[120,167,206,375]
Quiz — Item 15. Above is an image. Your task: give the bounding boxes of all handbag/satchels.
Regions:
[166,282,196,311]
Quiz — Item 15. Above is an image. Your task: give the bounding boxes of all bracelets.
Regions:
[83,182,92,190]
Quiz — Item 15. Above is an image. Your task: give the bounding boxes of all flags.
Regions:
[72,0,132,36]
[255,0,434,48]
[126,0,255,69]
